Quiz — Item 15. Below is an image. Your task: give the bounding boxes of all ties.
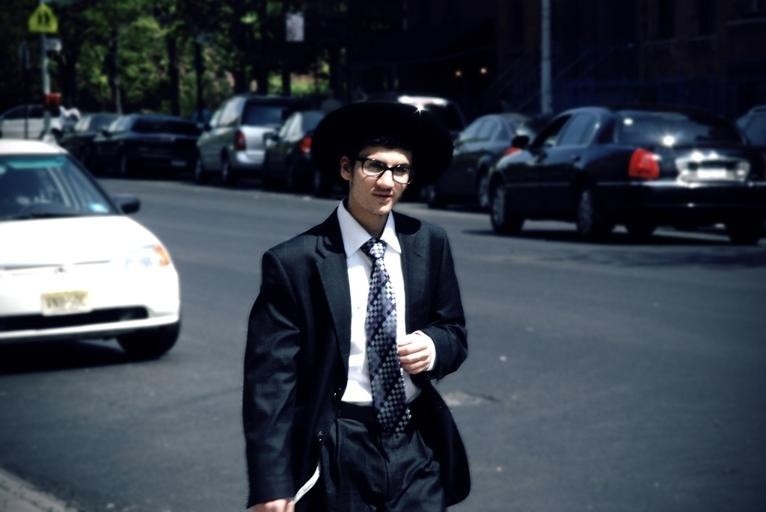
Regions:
[360,237,414,436]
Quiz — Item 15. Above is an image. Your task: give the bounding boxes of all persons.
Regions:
[240,97,474,512]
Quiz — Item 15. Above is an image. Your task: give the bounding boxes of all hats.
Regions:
[310,99,455,195]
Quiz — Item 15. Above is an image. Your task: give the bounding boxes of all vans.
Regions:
[384,94,465,144]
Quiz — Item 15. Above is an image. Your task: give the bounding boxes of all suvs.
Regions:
[196,92,293,184]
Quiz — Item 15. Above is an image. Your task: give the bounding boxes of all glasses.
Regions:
[349,155,418,187]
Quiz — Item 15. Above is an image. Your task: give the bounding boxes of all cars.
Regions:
[485,106,765,243]
[0,141,181,360]
[0,104,79,143]
[736,106,766,170]
[262,108,330,196]
[63,113,117,172]
[427,111,533,209]
[96,114,194,178]
